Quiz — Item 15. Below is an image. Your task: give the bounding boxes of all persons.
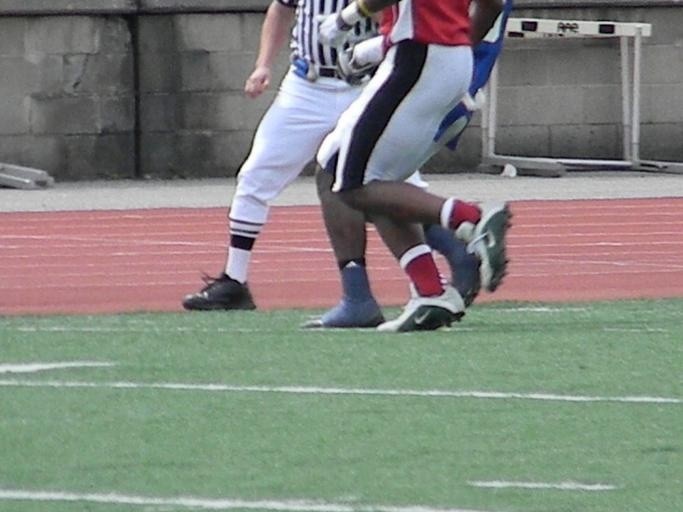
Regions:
[299,0,514,334]
[182,0,379,310]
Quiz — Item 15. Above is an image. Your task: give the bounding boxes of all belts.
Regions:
[315,67,368,81]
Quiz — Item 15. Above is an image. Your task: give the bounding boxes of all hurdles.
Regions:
[480,18,683,172]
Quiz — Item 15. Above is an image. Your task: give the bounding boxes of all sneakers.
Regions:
[455,202,514,293]
[180,270,257,310]
[447,239,482,309]
[298,298,385,329]
[376,278,466,332]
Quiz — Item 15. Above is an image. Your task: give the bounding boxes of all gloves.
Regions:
[311,2,367,50]
[333,36,386,83]
